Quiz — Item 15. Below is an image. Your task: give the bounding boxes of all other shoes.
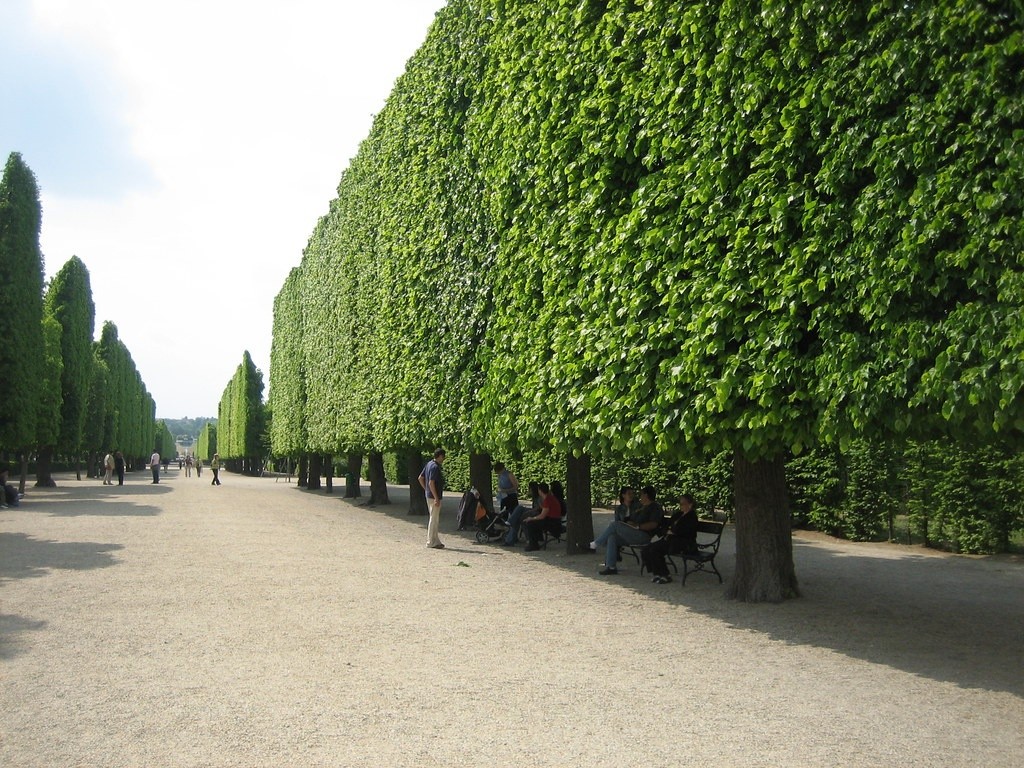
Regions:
[499,542,513,546]
[151,482,158,484]
[651,575,673,583]
[432,544,445,549]
[217,483,221,485]
[103,483,107,485]
[212,483,215,485]
[525,545,539,551]
[108,483,114,485]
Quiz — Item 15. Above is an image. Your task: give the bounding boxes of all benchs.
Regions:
[604,511,729,589]
[0,486,23,509]
[515,506,568,549]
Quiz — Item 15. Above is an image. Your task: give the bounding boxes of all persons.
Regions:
[418,448,446,548]
[0,446,9,508]
[499,481,567,552]
[641,494,698,584]
[494,462,520,525]
[150,449,160,484]
[103,450,126,485]
[210,454,221,485]
[179,455,202,477]
[576,485,665,575]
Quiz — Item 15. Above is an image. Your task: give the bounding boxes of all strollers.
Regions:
[464,491,510,544]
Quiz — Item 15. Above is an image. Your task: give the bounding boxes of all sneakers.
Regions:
[599,566,617,575]
[575,541,596,554]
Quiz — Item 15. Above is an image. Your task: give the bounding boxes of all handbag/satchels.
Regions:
[105,465,112,470]
